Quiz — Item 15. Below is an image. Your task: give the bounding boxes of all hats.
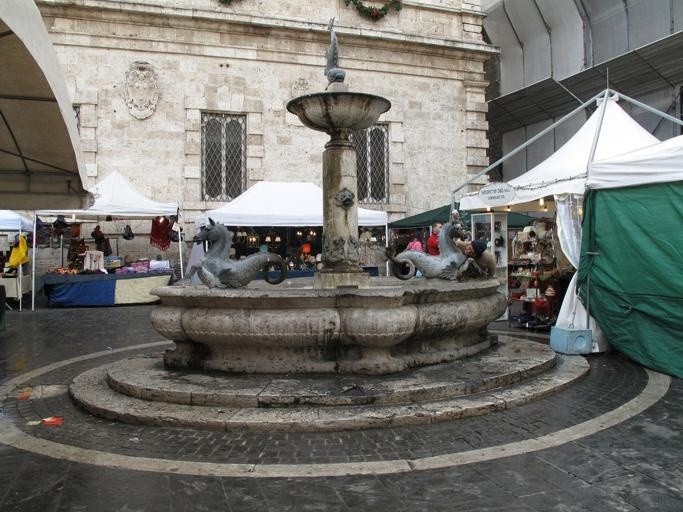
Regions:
[471,240,487,256]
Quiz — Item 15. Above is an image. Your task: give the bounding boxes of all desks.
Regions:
[44,270,173,310]
[0,274,31,311]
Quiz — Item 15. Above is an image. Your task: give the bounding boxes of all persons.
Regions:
[427,222,442,255]
[456,239,496,275]
[407,230,423,253]
